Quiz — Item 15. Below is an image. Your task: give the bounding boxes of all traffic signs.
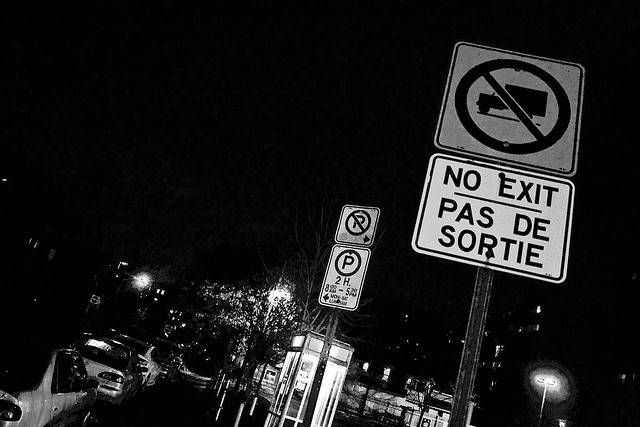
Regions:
[411,154,576,285]
[318,244,371,312]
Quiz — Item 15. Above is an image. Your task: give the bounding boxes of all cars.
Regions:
[111,333,163,388]
[70,333,143,405]
[0,343,99,427]
